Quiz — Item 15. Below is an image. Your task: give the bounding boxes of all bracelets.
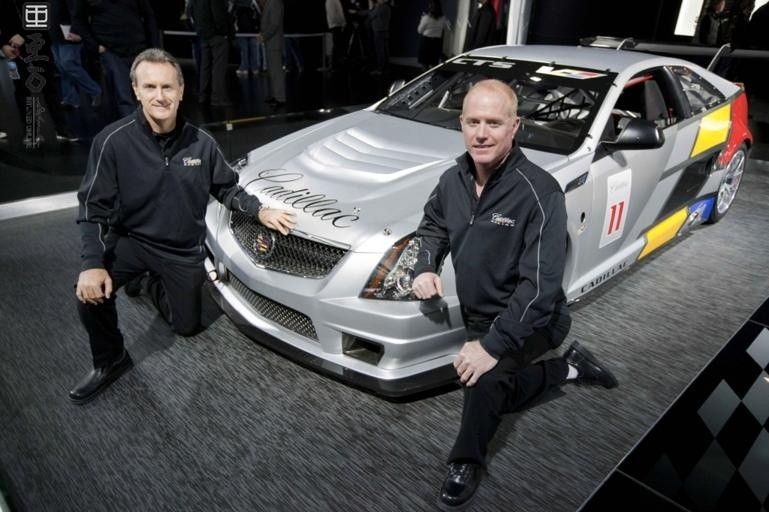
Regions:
[257,204,269,214]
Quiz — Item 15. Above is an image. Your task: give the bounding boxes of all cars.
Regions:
[205,35,754,400]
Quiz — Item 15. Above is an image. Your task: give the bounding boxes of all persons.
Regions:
[69,47,296,405]
[412,79,619,512]
[698,0,769,51]
[0,0,445,161]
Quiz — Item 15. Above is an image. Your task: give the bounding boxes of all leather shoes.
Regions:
[69,349,132,406]
[198,99,233,106]
[125,275,145,298]
[263,98,286,107]
[564,341,618,391]
[437,462,479,511]
